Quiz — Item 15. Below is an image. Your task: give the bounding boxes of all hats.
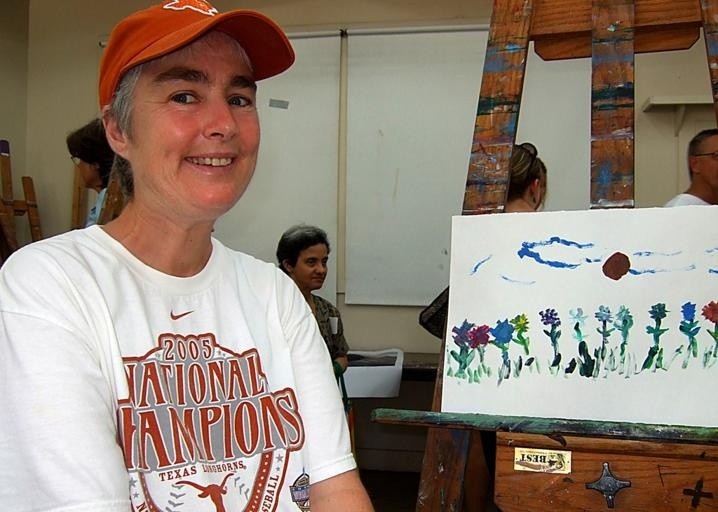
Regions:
[94,0,297,114]
[64,117,115,166]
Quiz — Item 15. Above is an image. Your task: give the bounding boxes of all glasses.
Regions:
[696,150,718,159]
[517,141,538,189]
[70,155,81,164]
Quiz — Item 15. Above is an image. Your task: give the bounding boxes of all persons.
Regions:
[662,128,718,207]
[0,0,375,512]
[65,118,116,228]
[503,142,547,213]
[276,225,350,379]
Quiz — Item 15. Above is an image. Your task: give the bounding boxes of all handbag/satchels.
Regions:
[338,370,357,462]
[419,285,450,339]
